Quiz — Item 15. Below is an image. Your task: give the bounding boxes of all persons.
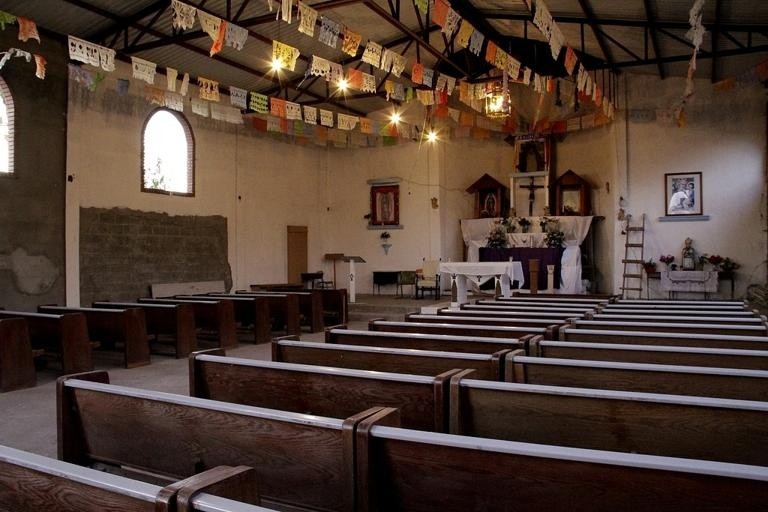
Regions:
[682,182,694,209]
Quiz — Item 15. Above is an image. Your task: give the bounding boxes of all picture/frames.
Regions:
[371,185,399,225]
[665,172,702,216]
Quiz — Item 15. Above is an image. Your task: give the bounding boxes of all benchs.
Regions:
[571,318,768,337]
[459,302,601,313]
[504,349,768,402]
[266,288,349,329]
[57,370,386,512]
[236,290,328,334]
[451,369,768,466]
[1,318,45,393]
[176,294,276,346]
[1,445,235,511]
[0,307,101,376]
[367,318,558,340]
[586,310,767,325]
[404,312,578,328]
[93,300,202,359]
[529,335,768,370]
[188,348,463,433]
[271,335,513,381]
[138,296,240,356]
[325,325,537,355]
[437,306,593,319]
[598,307,760,318]
[496,294,610,304]
[178,466,280,511]
[208,292,305,336]
[614,297,749,306]
[559,325,768,350]
[512,292,615,299]
[475,298,601,307]
[356,408,768,512]
[601,303,749,312]
[38,304,157,369]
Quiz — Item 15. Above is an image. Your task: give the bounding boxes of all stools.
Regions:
[302,274,324,288]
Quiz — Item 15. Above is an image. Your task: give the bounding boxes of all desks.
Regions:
[661,270,718,301]
[441,262,522,302]
[717,272,734,299]
[372,269,414,297]
[647,272,661,299]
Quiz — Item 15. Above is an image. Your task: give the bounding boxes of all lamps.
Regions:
[486,81,511,119]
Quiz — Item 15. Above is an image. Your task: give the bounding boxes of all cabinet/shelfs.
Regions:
[460,216,604,292]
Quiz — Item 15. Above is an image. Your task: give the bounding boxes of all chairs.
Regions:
[415,257,443,300]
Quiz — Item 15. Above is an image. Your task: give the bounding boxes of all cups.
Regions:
[447,257,452,263]
[509,257,514,263]
